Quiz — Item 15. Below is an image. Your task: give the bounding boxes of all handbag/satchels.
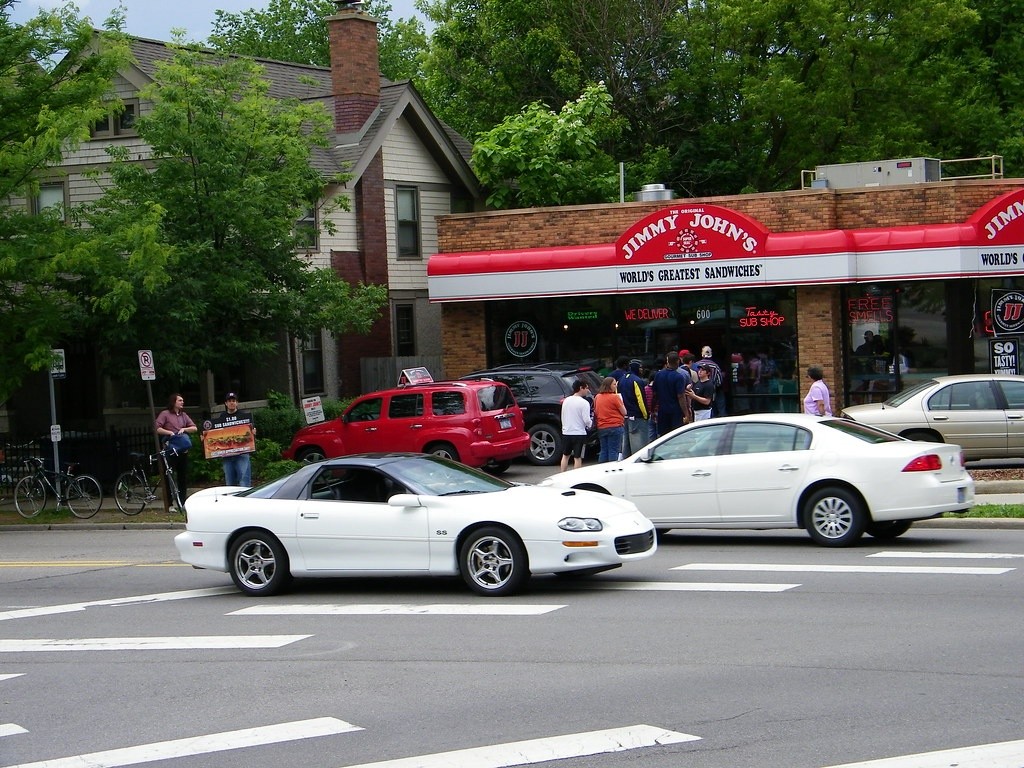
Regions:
[162,431,192,453]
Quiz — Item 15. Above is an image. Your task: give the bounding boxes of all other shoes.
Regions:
[169,506,177,512]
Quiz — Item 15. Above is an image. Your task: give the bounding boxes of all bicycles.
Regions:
[0,440,37,491]
[113,448,184,516]
[14,456,103,519]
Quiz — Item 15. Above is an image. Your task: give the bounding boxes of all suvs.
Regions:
[288,378,530,473]
[451,361,607,465]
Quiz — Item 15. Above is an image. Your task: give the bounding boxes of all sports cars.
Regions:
[174,449,658,597]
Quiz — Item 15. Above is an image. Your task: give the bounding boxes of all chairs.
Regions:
[333,477,396,502]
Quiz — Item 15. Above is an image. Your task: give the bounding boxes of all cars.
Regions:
[839,374,1024,460]
[534,413,976,547]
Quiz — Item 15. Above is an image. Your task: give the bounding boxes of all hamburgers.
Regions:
[205,426,253,450]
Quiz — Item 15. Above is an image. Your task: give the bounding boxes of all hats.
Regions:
[701,346,712,356]
[679,350,694,357]
[697,363,710,373]
[225,392,237,401]
[731,354,743,362]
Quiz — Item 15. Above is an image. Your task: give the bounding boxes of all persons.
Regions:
[803,367,833,449]
[199,393,256,488]
[730,348,799,412]
[154,393,197,512]
[852,331,908,403]
[560,346,725,472]
